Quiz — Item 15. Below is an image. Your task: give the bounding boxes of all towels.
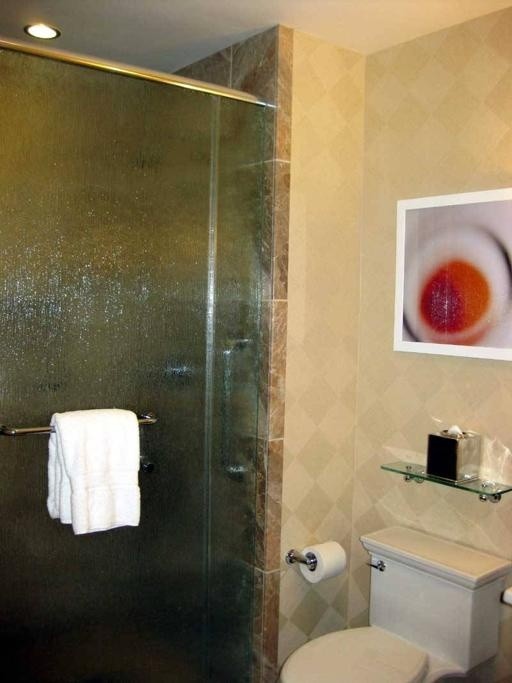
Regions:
[45,408,141,534]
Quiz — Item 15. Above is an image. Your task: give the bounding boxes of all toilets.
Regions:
[278,523,510,683]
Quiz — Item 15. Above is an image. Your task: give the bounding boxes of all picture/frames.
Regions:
[393,188,512,362]
